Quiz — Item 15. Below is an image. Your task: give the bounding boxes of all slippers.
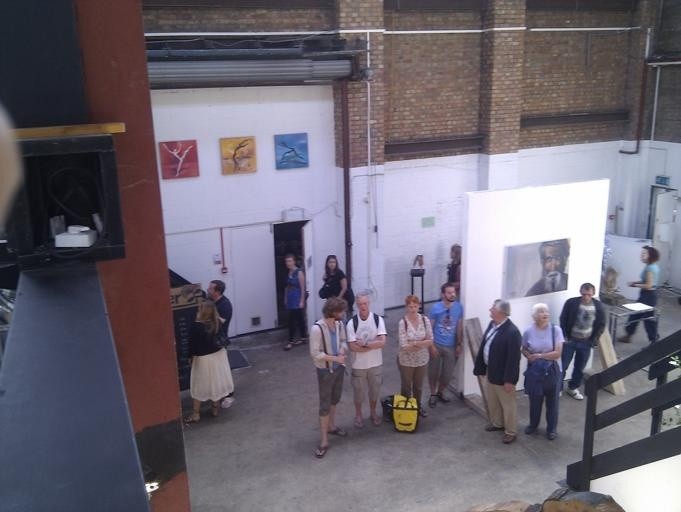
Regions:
[328,425,348,437]
[314,441,330,458]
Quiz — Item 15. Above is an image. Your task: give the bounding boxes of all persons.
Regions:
[183,298,235,425]
[447,244,461,303]
[557,283,607,401]
[346,293,388,429]
[523,240,571,298]
[206,280,235,410]
[618,245,661,348]
[0,105,26,238]
[284,255,308,350]
[396,295,435,418]
[310,297,349,458]
[426,284,464,407]
[520,303,566,440]
[319,255,354,329]
[473,299,522,444]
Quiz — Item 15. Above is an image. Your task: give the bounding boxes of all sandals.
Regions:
[353,414,382,429]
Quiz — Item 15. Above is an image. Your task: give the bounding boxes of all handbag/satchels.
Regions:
[543,360,565,398]
[380,392,421,434]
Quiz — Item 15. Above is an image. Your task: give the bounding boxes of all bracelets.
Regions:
[539,353,541,358]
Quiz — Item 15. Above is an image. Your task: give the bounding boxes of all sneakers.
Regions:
[184,414,200,424]
[617,335,632,343]
[284,337,310,350]
[525,426,535,434]
[546,432,558,440]
[485,422,501,432]
[418,390,452,417]
[209,405,222,417]
[502,434,516,444]
[565,386,583,400]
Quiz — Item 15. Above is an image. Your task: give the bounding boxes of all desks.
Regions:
[608,301,658,348]
[410,268,425,314]
